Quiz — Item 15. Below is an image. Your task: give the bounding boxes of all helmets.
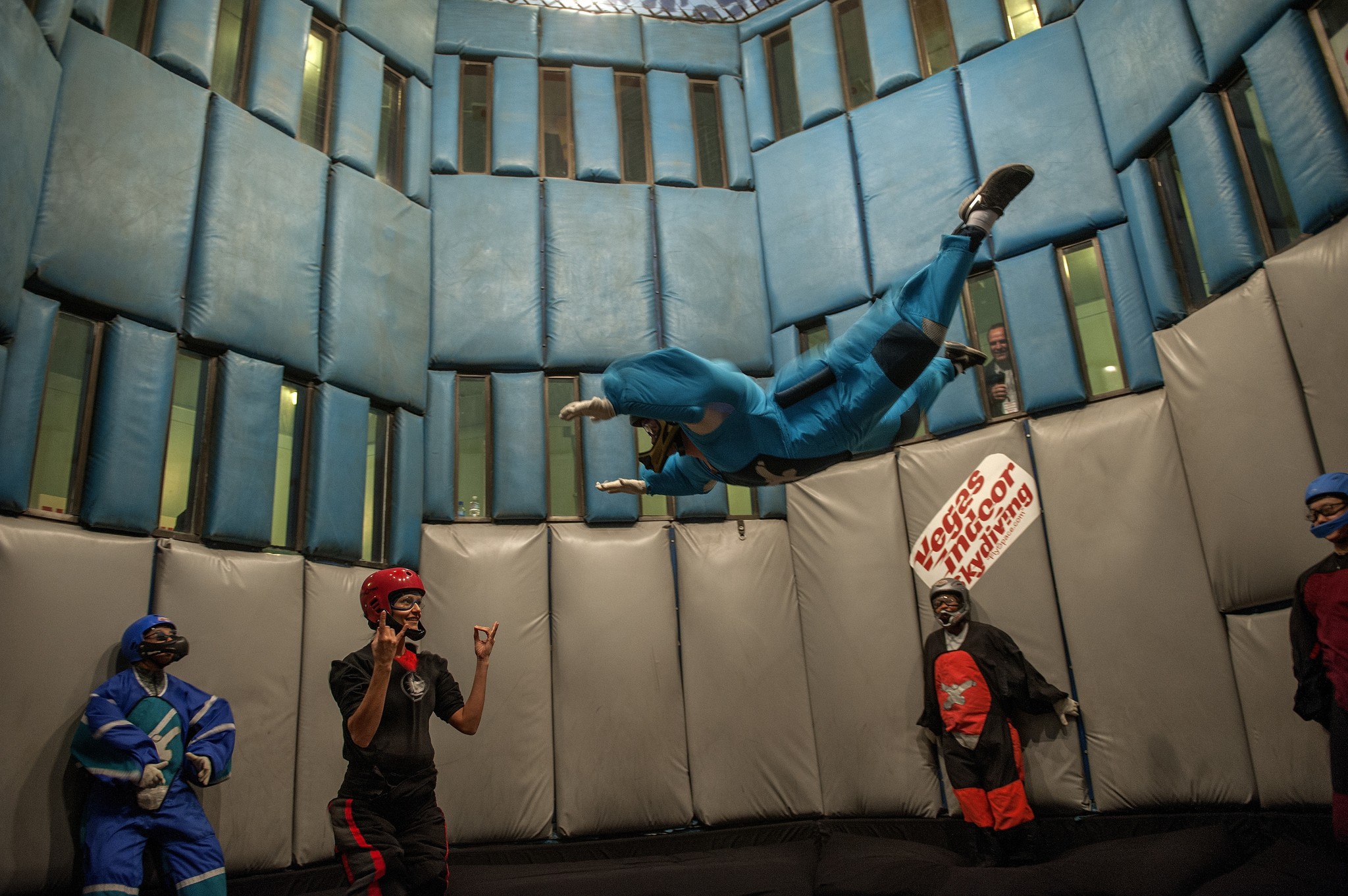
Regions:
[360,567,427,641]
[629,415,680,473]
[121,615,189,668]
[1306,473,1348,538]
[929,578,970,628]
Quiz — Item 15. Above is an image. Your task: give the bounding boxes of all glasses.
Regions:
[931,594,961,612]
[1305,501,1348,523]
[144,632,177,642]
[390,591,426,611]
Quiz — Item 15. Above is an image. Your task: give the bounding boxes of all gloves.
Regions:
[925,727,941,746]
[1052,697,1080,726]
[185,752,212,785]
[595,478,647,495]
[558,396,618,423]
[138,761,169,789]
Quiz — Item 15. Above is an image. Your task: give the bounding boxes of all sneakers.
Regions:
[944,341,988,371]
[958,162,1035,223]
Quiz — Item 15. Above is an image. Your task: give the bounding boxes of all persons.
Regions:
[915,578,1079,867]
[559,164,1035,497]
[327,568,499,896]
[70,615,235,896]
[983,322,1019,417]
[1290,473,1348,870]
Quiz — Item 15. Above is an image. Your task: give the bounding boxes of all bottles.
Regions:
[469,496,480,517]
[457,501,466,517]
